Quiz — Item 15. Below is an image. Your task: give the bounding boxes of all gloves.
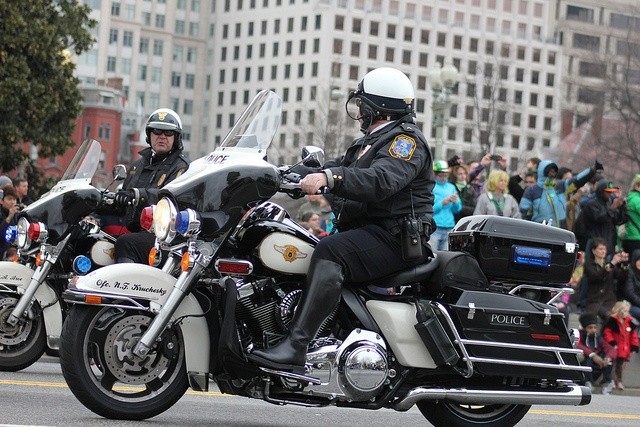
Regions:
[114,189,134,209]
[592,160,604,173]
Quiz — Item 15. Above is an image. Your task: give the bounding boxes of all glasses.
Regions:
[150,128,176,136]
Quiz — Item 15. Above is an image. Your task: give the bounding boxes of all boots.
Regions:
[246,258,344,376]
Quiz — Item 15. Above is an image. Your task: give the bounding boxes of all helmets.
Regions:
[145,108,183,150]
[346,67,415,132]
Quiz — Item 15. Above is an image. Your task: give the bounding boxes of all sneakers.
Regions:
[585,381,592,390]
[617,382,623,390]
[602,384,612,394]
[611,379,616,389]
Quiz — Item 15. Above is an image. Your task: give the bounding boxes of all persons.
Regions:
[427,154,640,254]
[247,67,437,375]
[295,194,338,237]
[0,175,34,263]
[617,250,640,322]
[578,236,629,322]
[575,313,617,394]
[603,300,640,390]
[115,107,191,267]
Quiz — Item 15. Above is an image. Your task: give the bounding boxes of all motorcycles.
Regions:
[0,138,137,372]
[58,89,595,427]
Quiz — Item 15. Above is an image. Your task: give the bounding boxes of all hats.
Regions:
[604,182,618,192]
[432,160,450,173]
[579,311,597,329]
